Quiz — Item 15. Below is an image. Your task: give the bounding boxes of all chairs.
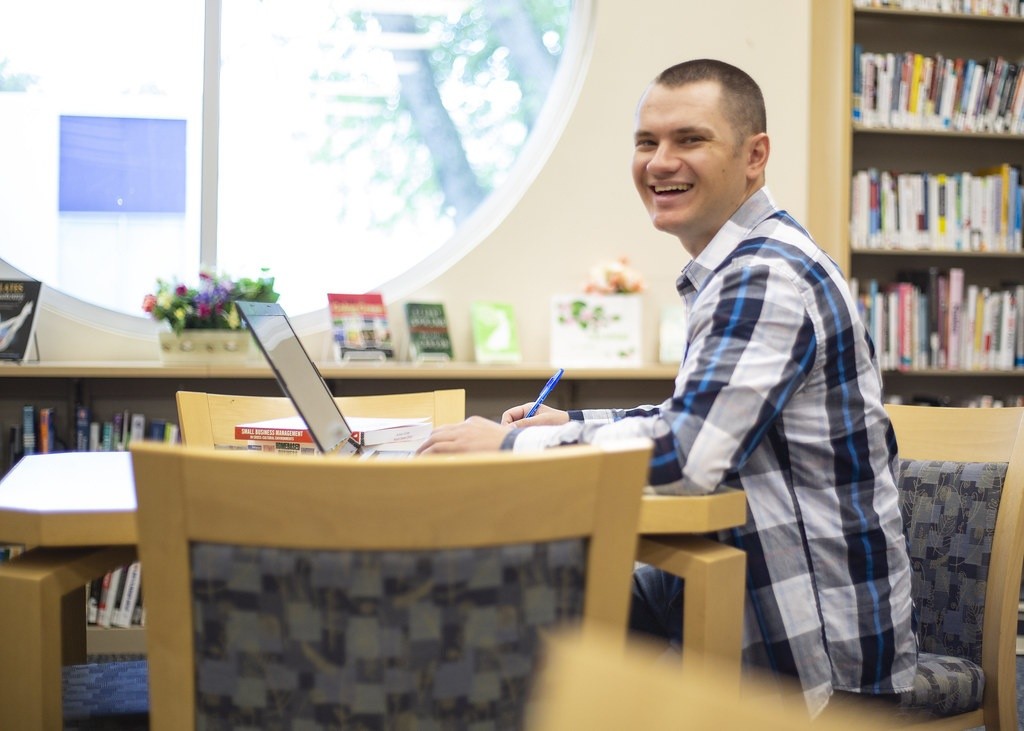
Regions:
[130,389,1024,731]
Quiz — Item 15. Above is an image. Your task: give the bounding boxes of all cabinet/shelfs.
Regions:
[0,363,678,657]
[808,0,1024,411]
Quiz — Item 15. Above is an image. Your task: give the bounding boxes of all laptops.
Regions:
[234,301,417,466]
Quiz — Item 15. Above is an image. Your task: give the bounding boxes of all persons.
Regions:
[416,58,917,721]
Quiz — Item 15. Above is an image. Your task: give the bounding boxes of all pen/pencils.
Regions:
[524,368,564,418]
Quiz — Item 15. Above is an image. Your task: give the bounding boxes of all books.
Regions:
[848,0,1024,407]
[0,403,177,630]
[473,303,522,363]
[327,293,395,361]
[405,303,454,361]
[0,279,42,362]
[235,416,434,455]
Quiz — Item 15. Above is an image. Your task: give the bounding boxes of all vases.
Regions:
[157,332,248,364]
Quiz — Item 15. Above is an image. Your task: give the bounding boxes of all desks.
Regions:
[0,452,748,731]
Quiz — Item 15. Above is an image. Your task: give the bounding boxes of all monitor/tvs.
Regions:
[58,114,186,213]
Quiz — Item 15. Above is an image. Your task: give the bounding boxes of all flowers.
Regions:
[142,270,281,329]
